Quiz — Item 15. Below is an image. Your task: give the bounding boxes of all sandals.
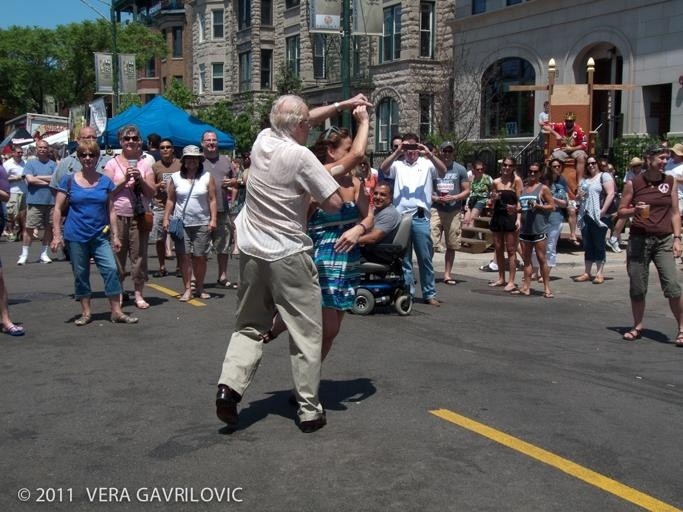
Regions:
[623,326,643,341]
[676,330,683,347]
[75,313,93,326]
[153,269,239,302]
[110,314,140,324]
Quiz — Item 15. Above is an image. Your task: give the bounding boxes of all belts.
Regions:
[116,215,136,221]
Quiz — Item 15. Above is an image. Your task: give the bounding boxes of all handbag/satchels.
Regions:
[598,171,620,215]
[137,211,154,233]
[59,174,72,217]
[167,217,186,243]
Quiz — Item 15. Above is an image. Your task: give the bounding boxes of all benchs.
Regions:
[457,204,494,254]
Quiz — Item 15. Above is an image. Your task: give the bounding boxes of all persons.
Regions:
[1,93,683,433]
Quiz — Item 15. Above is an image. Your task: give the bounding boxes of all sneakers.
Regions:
[605,239,622,254]
[40,254,53,263]
[16,255,29,265]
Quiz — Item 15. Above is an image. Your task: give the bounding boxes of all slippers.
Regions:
[443,278,457,285]
[487,276,555,299]
[479,260,500,272]
[2,323,26,336]
[134,298,149,309]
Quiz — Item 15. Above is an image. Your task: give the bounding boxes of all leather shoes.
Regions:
[214,384,239,427]
[574,273,592,282]
[592,275,604,284]
[300,411,327,435]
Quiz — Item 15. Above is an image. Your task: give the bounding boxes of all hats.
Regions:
[669,142,683,159]
[564,111,577,121]
[441,141,454,150]
[646,143,670,156]
[180,145,206,163]
[629,157,644,166]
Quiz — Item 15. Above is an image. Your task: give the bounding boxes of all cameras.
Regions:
[403,144,417,150]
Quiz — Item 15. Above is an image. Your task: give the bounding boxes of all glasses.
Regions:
[529,170,539,174]
[79,153,98,159]
[587,162,597,166]
[160,145,172,150]
[442,149,453,154]
[122,135,139,142]
[323,125,340,140]
[552,165,561,169]
[503,164,513,169]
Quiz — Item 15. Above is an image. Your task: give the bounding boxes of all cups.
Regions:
[640,204,651,218]
[507,204,514,214]
[127,160,138,169]
[441,190,449,197]
[55,244,66,261]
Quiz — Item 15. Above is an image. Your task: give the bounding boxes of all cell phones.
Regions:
[129,160,137,169]
